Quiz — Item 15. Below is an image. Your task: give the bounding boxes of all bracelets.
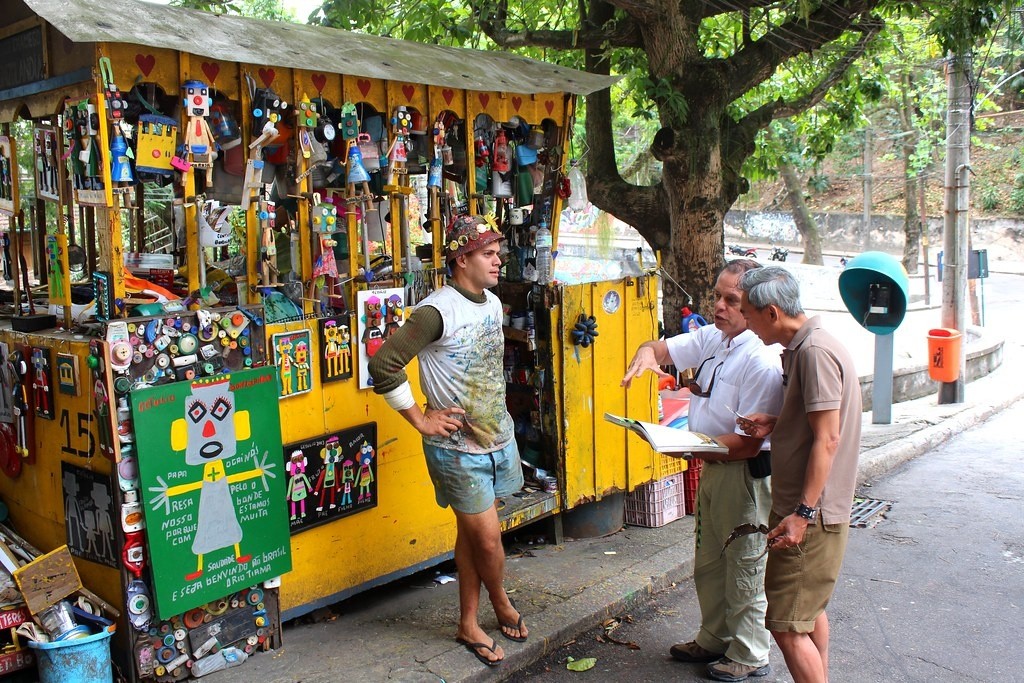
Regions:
[795,503,817,519]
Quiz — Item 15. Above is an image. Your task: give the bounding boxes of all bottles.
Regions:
[536,222,554,284]
[568,159,588,211]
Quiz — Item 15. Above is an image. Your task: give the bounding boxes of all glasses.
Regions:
[689,356,724,398]
[721,522,775,562]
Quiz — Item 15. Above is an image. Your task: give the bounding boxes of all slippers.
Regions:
[455,636,501,665]
[499,597,528,642]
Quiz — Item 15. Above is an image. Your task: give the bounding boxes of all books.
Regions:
[604,412,730,453]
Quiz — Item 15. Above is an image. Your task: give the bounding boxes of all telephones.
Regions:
[869,283,889,313]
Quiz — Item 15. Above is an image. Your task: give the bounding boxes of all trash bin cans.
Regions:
[926,327,962,383]
[28,623,116,683]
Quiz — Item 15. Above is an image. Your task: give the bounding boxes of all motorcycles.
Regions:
[768,243,791,262]
[725,242,759,259]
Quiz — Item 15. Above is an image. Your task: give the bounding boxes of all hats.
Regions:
[445,215,505,264]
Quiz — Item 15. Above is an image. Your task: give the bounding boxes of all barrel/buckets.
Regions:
[30,622,117,683]
[562,492,624,538]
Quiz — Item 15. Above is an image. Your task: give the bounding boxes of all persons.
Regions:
[620,260,784,682]
[736,266,862,683]
[367,215,529,666]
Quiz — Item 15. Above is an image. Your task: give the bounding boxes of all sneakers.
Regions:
[705,656,771,681]
[670,641,725,662]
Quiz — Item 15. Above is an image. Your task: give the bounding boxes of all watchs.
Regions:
[682,452,693,460]
